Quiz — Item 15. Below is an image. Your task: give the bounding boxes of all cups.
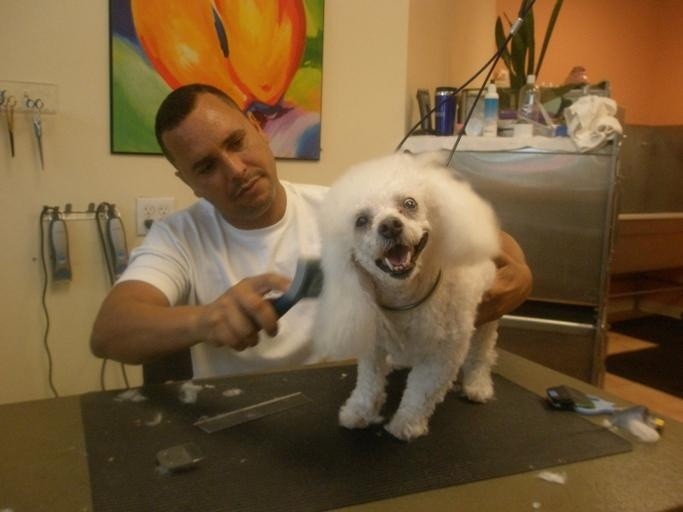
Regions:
[435,86,459,135]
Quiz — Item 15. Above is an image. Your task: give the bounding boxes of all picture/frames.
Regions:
[108,0,324,162]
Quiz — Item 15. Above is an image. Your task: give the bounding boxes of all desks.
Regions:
[0,345,682,512]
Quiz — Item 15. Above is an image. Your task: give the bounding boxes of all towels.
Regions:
[564,97,622,153]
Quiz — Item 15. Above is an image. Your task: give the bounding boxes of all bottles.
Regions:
[512,74,540,140]
[483,79,499,137]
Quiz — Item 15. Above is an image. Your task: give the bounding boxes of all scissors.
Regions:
[546,383,648,417]
[27,98,47,169]
[1,90,18,159]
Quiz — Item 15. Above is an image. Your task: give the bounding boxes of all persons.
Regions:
[89,81,531,379]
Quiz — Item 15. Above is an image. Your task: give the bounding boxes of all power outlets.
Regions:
[134,196,176,238]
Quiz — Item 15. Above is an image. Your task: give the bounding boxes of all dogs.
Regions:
[313,149,506,443]
